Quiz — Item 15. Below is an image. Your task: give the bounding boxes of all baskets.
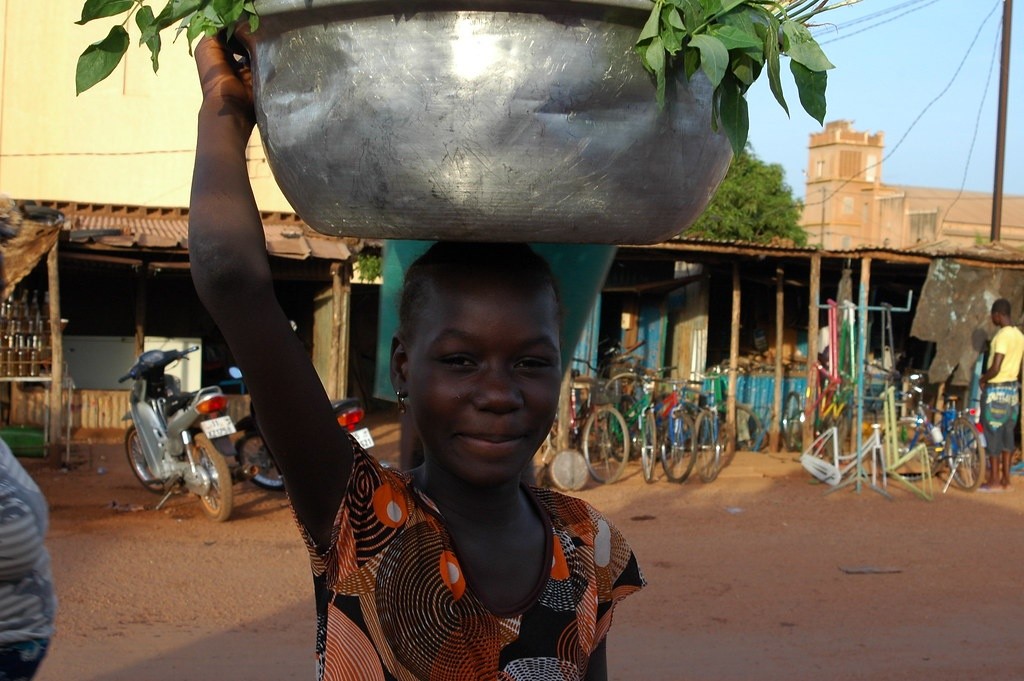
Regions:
[591,378,620,405]
[130,375,180,406]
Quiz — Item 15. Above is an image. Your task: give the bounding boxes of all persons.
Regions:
[816,327,830,386]
[0,438,58,681]
[977,298,1024,490]
[187,21,646,681]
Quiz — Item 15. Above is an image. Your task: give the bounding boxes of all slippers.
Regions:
[976,483,1014,493]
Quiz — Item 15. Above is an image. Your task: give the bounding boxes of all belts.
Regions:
[986,381,1018,386]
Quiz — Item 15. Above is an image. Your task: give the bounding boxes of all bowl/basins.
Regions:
[231,0,736,244]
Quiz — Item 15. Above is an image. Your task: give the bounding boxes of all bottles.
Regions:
[0,288,45,378]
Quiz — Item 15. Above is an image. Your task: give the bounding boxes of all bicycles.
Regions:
[876,371,988,493]
[783,365,848,456]
[552,338,767,487]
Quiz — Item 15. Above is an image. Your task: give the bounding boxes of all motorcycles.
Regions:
[117,345,237,524]
[229,320,374,493]
